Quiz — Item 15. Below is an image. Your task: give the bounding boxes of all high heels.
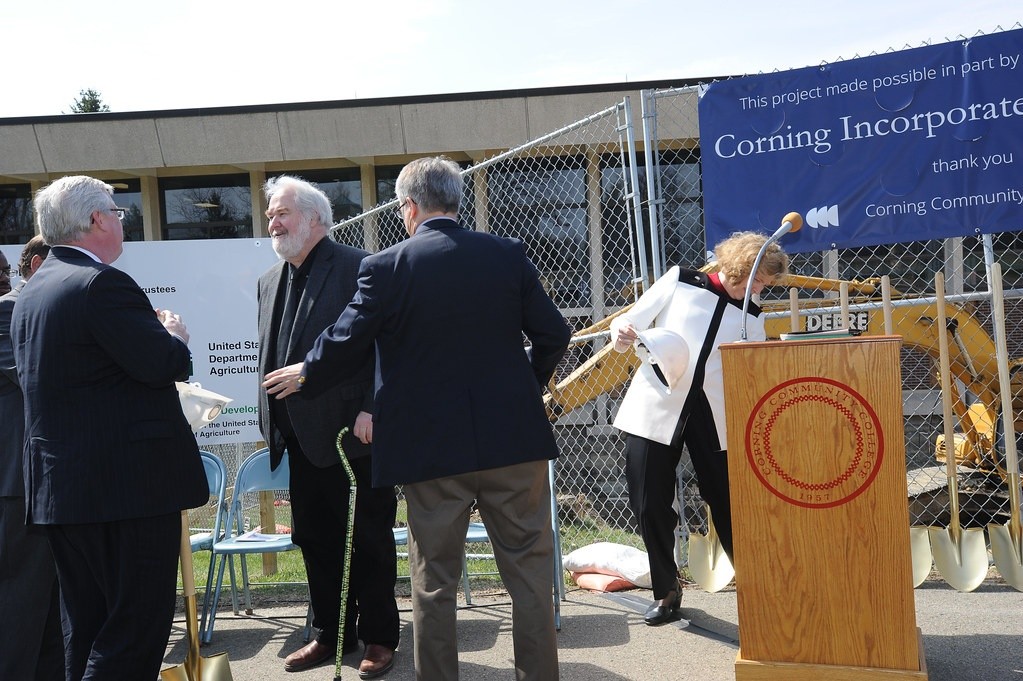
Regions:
[644,582,683,625]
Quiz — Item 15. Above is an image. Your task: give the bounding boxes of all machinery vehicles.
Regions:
[543,262,1023,526]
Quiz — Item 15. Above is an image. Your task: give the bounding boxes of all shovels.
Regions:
[687,506,735,593]
[927,272,987,593]
[986,263,1022,592]
[155,509,235,681]
[880,275,933,588]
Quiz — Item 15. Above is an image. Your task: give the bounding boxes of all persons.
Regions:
[609,229,789,627]
[0,174,211,681]
[256,175,401,678]
[262,155,572,681]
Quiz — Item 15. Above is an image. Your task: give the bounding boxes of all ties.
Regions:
[274,271,299,416]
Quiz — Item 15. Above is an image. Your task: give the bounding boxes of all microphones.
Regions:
[734,212,803,343]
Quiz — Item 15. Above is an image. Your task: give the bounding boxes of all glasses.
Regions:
[394,197,417,219]
[0,268,18,279]
[92,208,126,225]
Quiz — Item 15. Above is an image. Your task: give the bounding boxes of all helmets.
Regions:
[627,325,690,395]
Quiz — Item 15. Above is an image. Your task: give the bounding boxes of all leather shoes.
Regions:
[284,639,359,671]
[358,644,394,678]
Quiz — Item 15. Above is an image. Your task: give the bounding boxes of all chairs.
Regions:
[462,462,565,631]
[190,451,238,647]
[205,447,316,644]
